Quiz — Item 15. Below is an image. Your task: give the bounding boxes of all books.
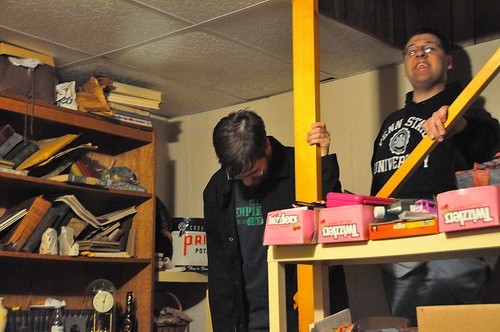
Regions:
[49,174,107,188]
[5,309,94,332]
[104,80,163,130]
[0,193,137,259]
[0,160,28,176]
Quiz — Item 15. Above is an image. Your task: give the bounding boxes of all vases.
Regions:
[0,297,8,332]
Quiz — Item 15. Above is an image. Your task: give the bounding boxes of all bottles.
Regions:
[0,297,8,332]
[50,306,65,332]
[373,199,434,222]
[121,292,137,332]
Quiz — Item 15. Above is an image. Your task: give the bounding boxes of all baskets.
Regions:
[153,291,186,332]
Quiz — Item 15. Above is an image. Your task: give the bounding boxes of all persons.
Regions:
[369,28,500,326]
[203,110,342,332]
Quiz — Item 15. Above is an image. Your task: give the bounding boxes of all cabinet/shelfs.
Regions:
[267,225,500,332]
[0,92,207,332]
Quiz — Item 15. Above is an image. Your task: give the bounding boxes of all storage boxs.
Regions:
[369,218,438,239]
[263,204,376,246]
[436,185,500,232]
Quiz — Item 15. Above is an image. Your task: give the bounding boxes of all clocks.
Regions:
[92,290,115,332]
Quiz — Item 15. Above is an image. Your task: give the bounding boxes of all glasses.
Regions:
[227,158,266,180]
[405,44,448,57]
